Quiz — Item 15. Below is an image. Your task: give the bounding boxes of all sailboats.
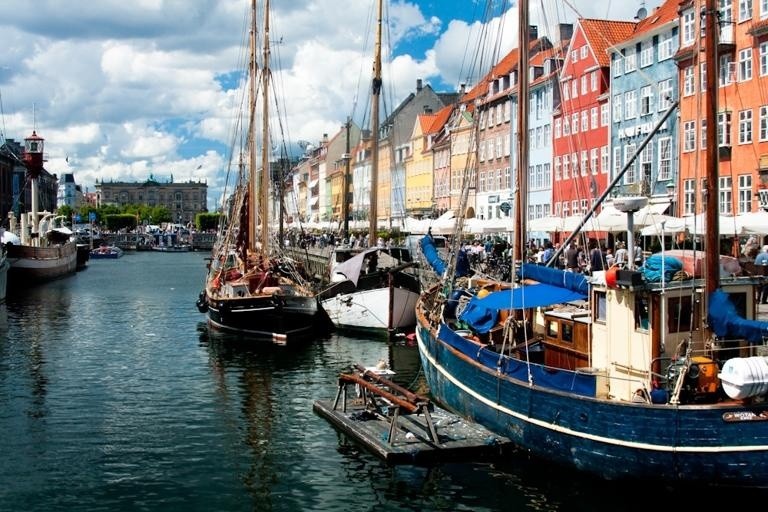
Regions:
[0,101,77,282]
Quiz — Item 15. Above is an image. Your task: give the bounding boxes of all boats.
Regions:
[89,246,123,258]
[0,249,9,303]
[136,239,153,250]
[153,243,197,252]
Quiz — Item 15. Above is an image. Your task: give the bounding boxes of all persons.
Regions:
[296,230,395,253]
[459,236,642,275]
[754,245,768,304]
